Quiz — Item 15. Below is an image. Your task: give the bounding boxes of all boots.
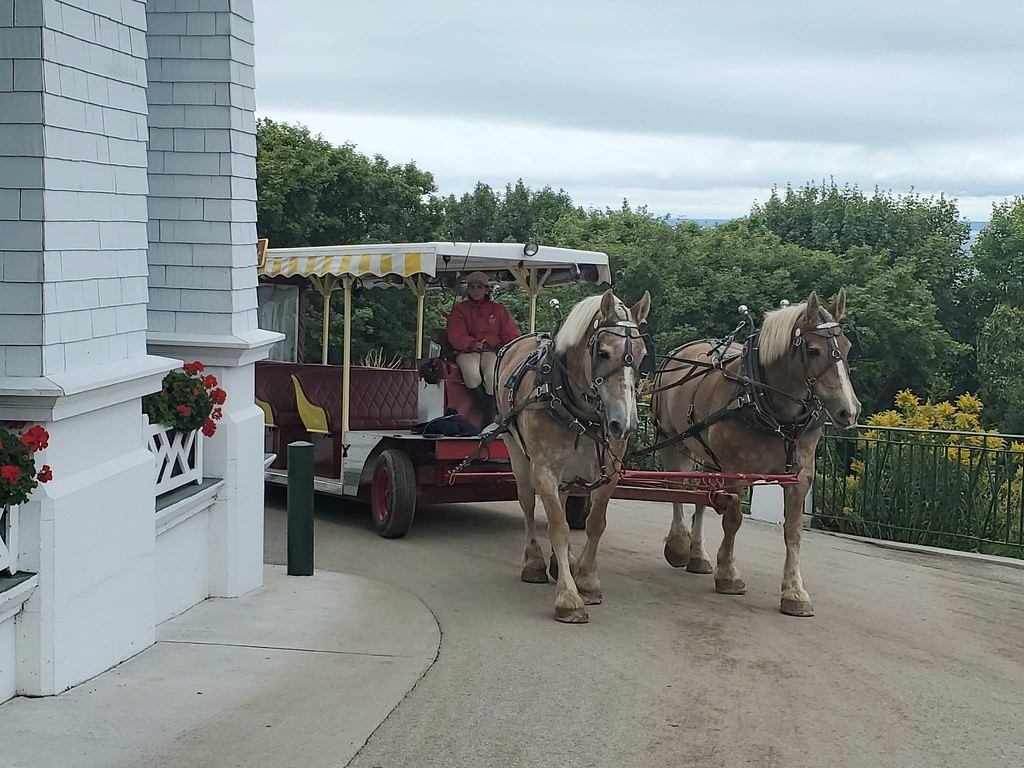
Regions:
[469,383,487,413]
[482,394,497,428]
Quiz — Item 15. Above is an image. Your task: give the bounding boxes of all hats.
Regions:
[467,272,488,285]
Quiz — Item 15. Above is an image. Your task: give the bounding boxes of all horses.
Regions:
[490,288,651,624]
[651,287,861,616]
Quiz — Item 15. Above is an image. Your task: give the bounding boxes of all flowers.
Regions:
[143,361,226,438]
[0,419,53,508]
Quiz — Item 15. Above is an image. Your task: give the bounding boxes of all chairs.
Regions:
[429,328,461,378]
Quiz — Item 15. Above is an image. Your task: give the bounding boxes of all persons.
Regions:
[447,271,519,427]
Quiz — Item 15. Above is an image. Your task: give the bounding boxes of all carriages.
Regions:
[254,239,862,626]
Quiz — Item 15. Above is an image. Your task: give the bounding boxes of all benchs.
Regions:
[254,361,419,479]
